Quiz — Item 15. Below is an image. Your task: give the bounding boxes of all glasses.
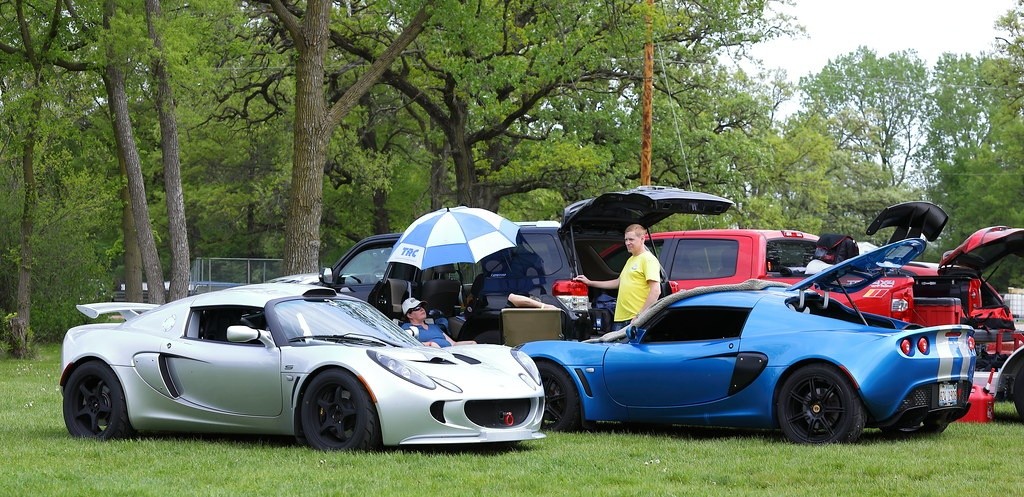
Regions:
[408,305,423,313]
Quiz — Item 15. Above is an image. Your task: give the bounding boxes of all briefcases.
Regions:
[556,295,589,312]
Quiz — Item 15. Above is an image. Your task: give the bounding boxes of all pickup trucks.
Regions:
[594,198,1024,422]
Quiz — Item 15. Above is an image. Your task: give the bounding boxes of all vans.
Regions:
[312,185,739,352]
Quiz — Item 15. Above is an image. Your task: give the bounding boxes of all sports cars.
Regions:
[57,279,549,456]
[512,235,979,448]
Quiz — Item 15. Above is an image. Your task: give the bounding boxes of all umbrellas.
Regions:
[386,205,520,273]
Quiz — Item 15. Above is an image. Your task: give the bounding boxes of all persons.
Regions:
[572,223,662,334]
[401,298,477,347]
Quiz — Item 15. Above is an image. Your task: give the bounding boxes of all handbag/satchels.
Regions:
[969,305,1015,331]
[567,292,616,342]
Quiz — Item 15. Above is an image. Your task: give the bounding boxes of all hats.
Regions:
[401,297,428,316]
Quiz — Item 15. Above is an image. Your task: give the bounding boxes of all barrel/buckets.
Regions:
[954,384,994,423]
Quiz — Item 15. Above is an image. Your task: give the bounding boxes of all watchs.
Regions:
[637,313,641,318]
